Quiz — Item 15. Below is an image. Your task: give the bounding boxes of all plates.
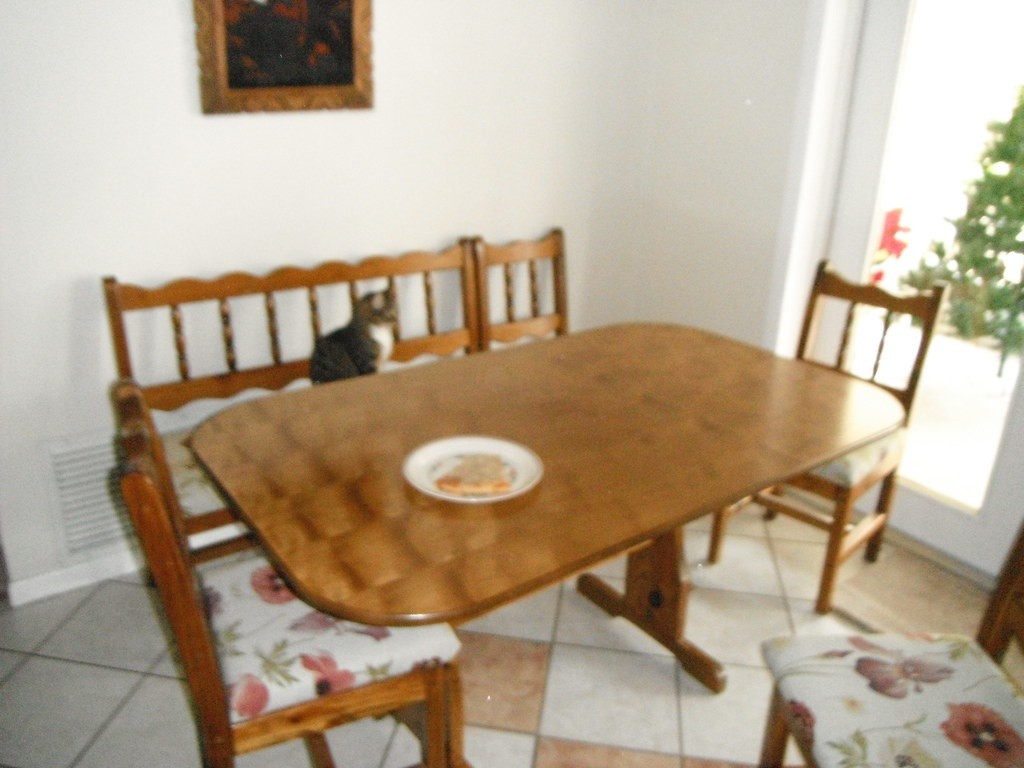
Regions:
[401,434,545,505]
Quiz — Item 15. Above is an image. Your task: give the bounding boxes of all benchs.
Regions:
[103,238,477,564]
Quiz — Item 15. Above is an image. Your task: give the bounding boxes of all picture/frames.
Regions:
[192,0,375,117]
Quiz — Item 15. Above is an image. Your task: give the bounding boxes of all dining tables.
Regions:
[188,320,907,744]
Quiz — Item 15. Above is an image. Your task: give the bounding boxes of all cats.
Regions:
[309,291,399,387]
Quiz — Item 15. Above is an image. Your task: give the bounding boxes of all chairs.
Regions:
[475,228,569,354]
[706,259,946,615]
[108,379,467,768]
[758,520,1024,768]
